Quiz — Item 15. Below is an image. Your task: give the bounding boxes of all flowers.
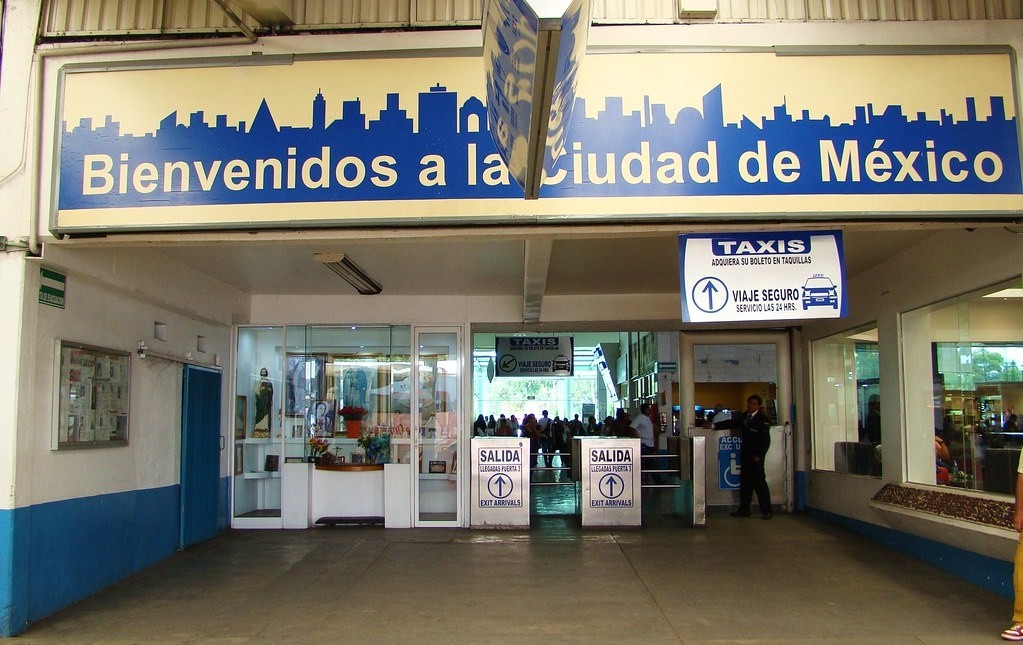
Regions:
[339,405,368,420]
[308,437,331,457]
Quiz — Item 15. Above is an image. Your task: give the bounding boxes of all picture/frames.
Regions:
[285,352,437,433]
[235,395,248,440]
[308,399,337,437]
[264,455,279,471]
[429,461,446,473]
[236,444,243,475]
[349,452,366,464]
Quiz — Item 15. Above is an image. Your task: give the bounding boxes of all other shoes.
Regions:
[546,462,550,469]
[729,508,751,518]
[762,512,772,520]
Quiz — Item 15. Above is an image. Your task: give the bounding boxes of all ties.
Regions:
[747,415,752,423]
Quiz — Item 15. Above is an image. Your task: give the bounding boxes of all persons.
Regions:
[861,393,882,479]
[1003,409,1018,441]
[701,395,772,520]
[253,368,274,438]
[473,408,633,470]
[695,404,730,427]
[629,403,667,484]
[935,436,950,486]
[674,410,680,436]
[1001,447,1023,641]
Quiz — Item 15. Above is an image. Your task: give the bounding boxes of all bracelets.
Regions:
[939,440,944,444]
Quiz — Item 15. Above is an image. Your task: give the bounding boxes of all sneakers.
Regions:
[1001,625,1023,640]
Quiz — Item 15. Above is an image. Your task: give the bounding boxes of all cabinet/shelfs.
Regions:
[245,437,458,483]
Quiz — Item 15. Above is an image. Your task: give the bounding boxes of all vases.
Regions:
[346,420,361,438]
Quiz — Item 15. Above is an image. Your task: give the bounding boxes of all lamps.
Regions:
[314,251,384,296]
